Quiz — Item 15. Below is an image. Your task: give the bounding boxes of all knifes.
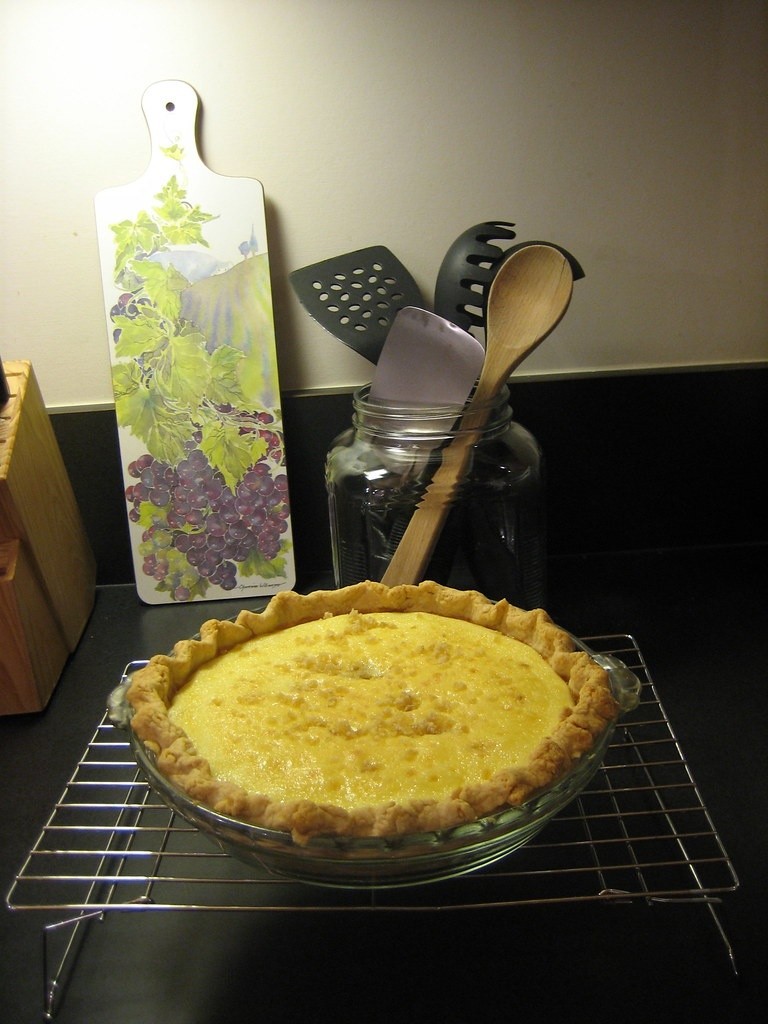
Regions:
[0,356,10,407]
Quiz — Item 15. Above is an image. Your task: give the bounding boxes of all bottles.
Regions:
[324,381,541,587]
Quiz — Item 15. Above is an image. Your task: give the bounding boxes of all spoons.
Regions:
[483,240,586,358]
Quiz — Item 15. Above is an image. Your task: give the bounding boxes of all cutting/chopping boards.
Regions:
[91,78,300,606]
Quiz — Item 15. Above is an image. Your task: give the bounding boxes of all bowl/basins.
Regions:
[115,593,640,892]
